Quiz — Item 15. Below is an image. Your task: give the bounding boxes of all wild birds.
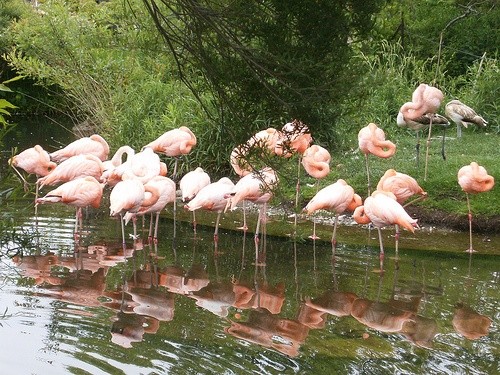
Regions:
[302,143,334,193]
[443,99,492,140]
[357,123,396,194]
[178,166,212,235]
[375,169,426,255]
[139,123,197,187]
[351,191,421,275]
[228,168,280,239]
[183,175,241,239]
[8,144,55,215]
[230,119,312,178]
[300,176,357,245]
[456,162,495,254]
[103,145,176,254]
[395,84,451,180]
[32,175,111,249]
[38,133,108,236]
